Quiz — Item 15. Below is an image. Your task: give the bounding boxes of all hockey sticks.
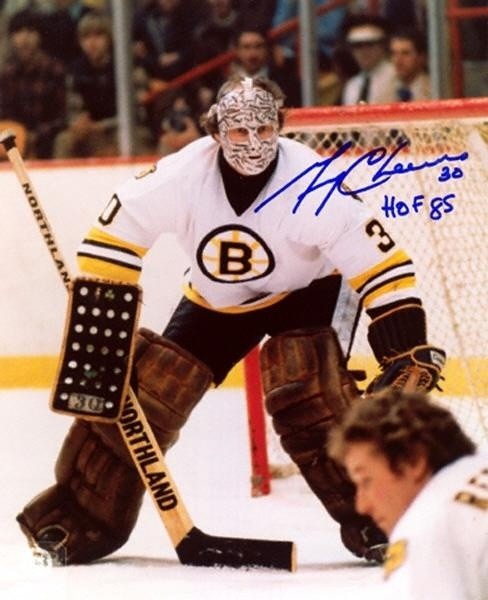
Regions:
[1,130,296,572]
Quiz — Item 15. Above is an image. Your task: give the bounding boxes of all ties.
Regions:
[357,76,370,104]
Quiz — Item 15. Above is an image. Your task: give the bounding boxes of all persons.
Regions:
[1,0,433,161]
[14,73,446,569]
[323,384,488,600]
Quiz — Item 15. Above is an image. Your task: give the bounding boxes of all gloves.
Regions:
[363,341,448,400]
